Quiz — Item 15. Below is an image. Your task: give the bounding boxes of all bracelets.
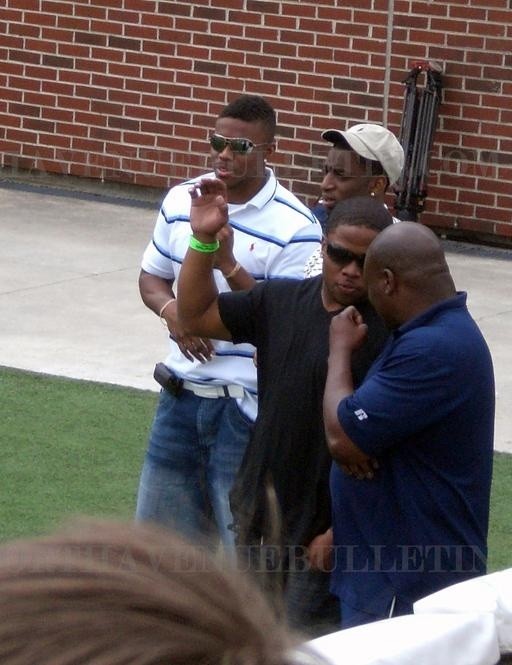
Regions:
[224,262,242,279]
[159,298,175,326]
[187,233,221,253]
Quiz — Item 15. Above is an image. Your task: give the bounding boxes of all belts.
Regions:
[182,379,244,398]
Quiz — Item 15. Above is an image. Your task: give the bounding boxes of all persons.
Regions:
[0,517,321,665]
[303,122,407,280]
[172,178,392,622]
[323,223,495,628]
[138,96,325,567]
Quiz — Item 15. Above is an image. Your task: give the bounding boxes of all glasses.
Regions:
[323,165,385,182]
[324,234,365,269]
[208,134,268,156]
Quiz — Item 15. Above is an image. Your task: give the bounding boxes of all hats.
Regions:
[321,123,405,189]
